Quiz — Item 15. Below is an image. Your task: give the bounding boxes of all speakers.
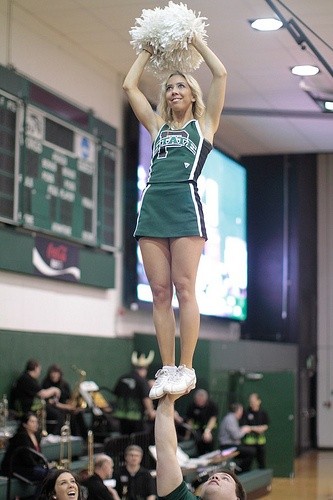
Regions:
[250,308,291,343]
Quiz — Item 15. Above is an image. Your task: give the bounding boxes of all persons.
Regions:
[121,36,228,399]
[0,351,271,500]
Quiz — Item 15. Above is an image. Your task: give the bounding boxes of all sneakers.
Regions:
[149,366,177,399]
[163,366,197,395]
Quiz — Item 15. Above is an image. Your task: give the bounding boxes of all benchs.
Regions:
[0,399,274,500]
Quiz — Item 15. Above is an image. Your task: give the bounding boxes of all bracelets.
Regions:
[143,49,153,56]
